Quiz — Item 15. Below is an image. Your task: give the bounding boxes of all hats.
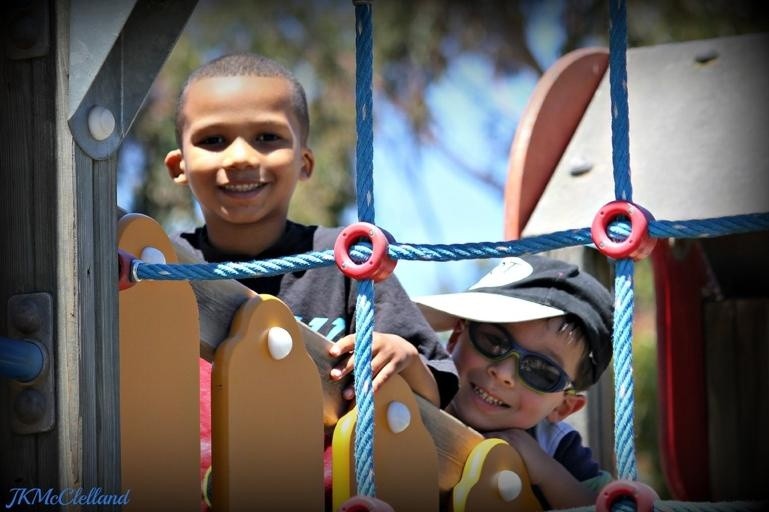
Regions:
[407,255,614,384]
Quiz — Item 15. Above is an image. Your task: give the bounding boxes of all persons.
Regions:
[165,54,459,409]
[405,254,614,510]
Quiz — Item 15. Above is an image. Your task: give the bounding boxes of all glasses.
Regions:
[467,320,584,396]
